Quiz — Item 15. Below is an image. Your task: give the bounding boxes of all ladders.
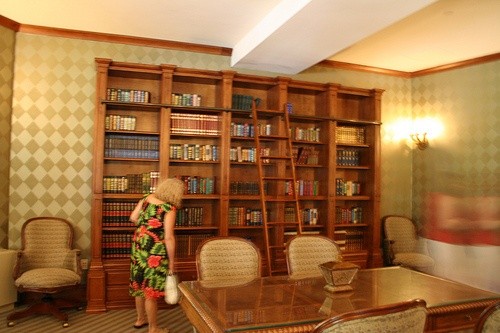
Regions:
[250,100,303,277]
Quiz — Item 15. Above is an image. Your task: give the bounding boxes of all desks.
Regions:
[176,264,499,332]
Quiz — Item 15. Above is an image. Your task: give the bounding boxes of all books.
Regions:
[336,124,366,251]
[101,87,160,259]
[169,91,218,256]
[284,100,319,254]
[228,93,272,242]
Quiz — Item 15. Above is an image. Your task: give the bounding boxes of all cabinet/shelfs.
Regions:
[88,56,384,314]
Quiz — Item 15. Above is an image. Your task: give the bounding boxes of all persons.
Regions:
[127,176,185,333]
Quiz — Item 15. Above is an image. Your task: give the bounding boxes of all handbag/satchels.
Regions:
[165,270,180,304]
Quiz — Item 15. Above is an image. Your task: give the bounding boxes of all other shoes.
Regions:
[133,318,172,333]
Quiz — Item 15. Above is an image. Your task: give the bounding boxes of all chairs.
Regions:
[381,215,435,274]
[8,216,87,329]
[312,297,430,333]
[282,232,344,281]
[194,236,261,284]
[473,303,499,332]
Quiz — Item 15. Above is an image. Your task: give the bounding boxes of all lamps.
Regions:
[400,115,439,148]
